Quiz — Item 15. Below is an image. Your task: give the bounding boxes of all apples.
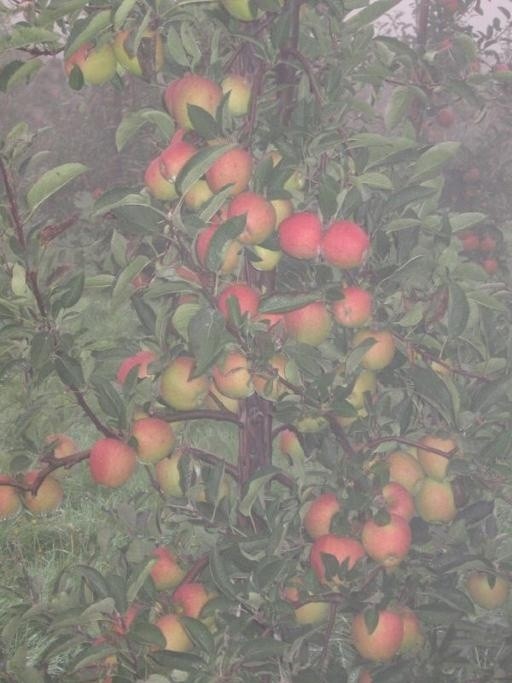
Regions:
[1,0,512,683]
